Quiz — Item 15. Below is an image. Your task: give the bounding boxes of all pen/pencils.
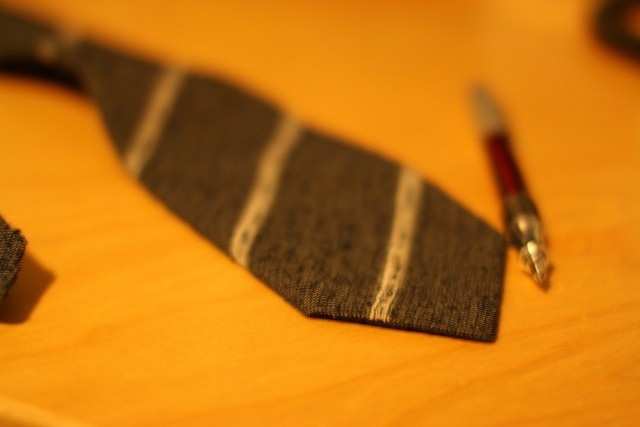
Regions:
[471,81,552,284]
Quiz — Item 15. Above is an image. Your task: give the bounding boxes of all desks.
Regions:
[1,0,640,427]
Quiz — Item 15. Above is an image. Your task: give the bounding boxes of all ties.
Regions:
[1,14,506,342]
